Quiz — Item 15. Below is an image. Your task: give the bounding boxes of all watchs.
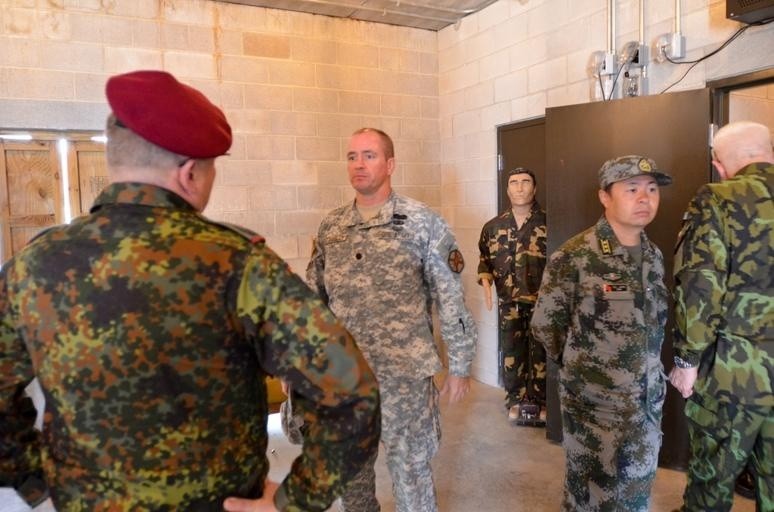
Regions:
[673,355,693,369]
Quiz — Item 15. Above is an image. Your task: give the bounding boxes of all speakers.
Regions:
[726,0,774,25]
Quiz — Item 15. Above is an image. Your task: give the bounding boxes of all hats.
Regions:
[599,155,672,189]
[107,71,232,158]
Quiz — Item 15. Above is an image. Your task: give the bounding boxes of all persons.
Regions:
[667,120,774,512]
[476,168,548,426]
[280,127,475,511]
[530,155,677,511]
[0,71,381,512]
